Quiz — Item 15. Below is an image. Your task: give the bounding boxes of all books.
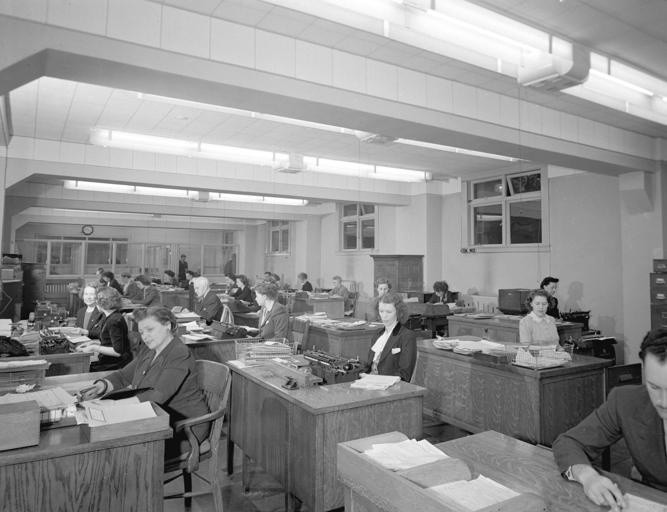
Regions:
[0,358,170,452]
[432,334,573,371]
[351,369,401,394]
[456,309,522,321]
[348,428,520,512]
[349,318,366,330]
[606,490,664,512]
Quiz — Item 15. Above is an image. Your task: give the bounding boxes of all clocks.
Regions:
[82,225,95,235]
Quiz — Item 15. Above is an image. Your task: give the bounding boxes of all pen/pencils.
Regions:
[615,483,623,511]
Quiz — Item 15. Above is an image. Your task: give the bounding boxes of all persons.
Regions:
[366,277,392,323]
[550,325,667,512]
[426,279,453,303]
[79,304,212,462]
[50,252,349,374]
[541,276,562,323]
[517,290,567,352]
[361,292,417,382]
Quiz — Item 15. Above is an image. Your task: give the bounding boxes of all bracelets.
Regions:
[562,466,577,483]
[93,378,109,396]
